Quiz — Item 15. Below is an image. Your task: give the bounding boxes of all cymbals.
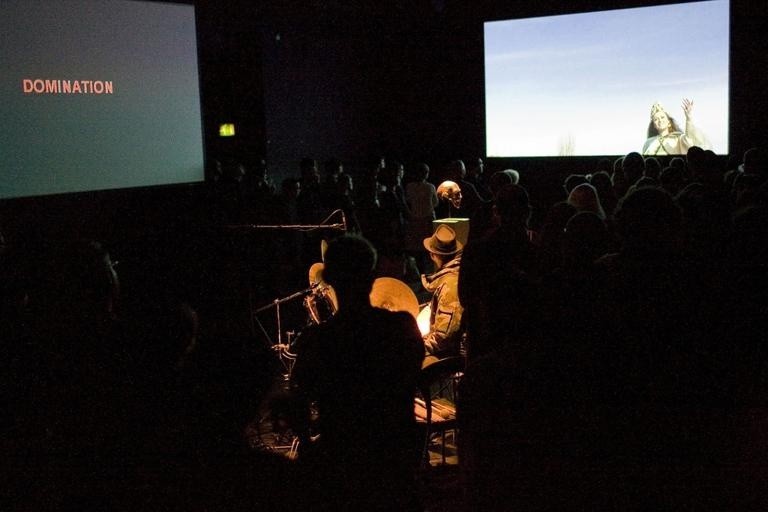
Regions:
[417,355,461,384]
[369,277,419,315]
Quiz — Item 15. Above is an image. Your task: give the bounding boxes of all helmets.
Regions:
[650,104,667,118]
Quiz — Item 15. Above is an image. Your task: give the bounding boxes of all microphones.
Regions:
[340,211,347,231]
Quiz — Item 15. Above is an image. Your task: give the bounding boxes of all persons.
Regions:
[641,99,704,154]
[0,146,766,512]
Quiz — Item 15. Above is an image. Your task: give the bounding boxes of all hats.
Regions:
[423,224,463,255]
[568,183,598,199]
